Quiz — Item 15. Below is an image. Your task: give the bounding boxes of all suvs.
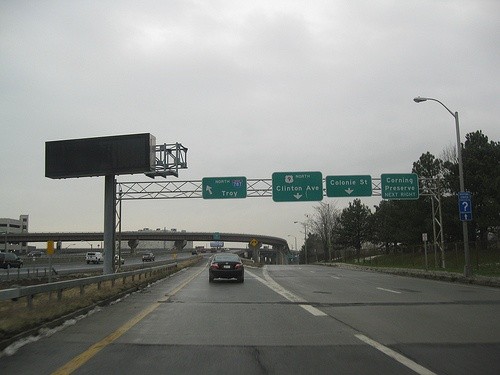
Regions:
[86,252,103,264]
[0,252,24,269]
[27,250,42,257]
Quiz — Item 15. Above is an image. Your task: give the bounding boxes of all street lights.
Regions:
[294,221,308,265]
[414,96,473,277]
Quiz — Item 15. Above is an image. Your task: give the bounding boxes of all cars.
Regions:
[142,253,155,262]
[208,253,246,284]
[192,247,230,255]
[114,255,125,265]
